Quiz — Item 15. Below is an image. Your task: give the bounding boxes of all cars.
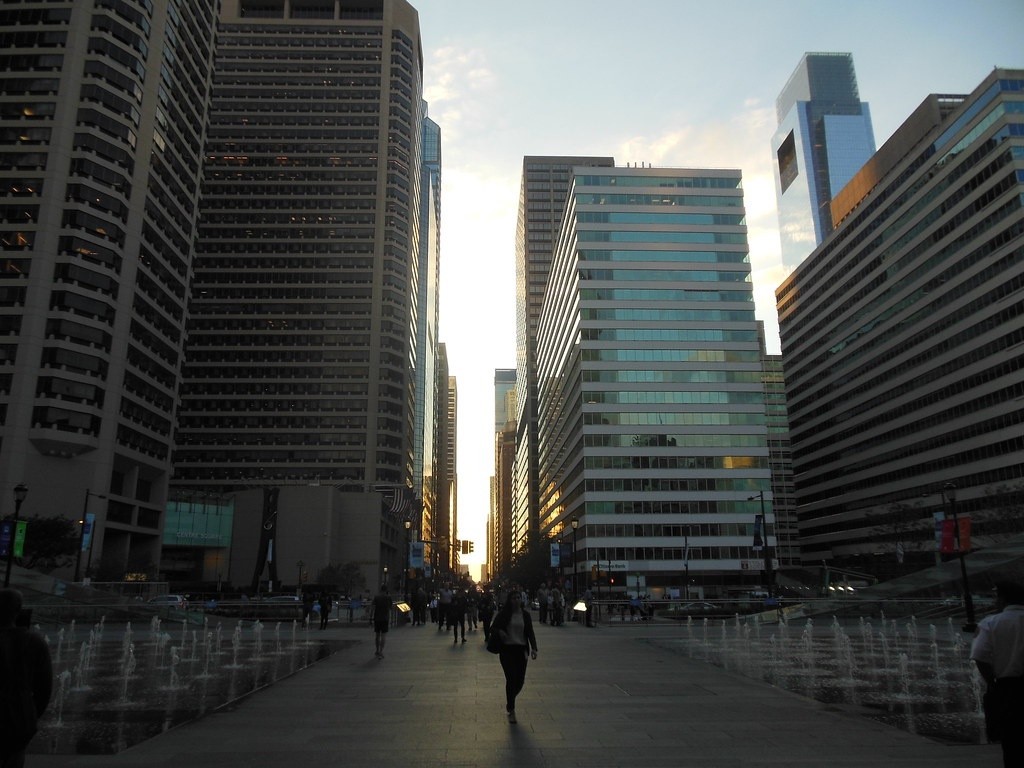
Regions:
[531,598,540,611]
[340,595,352,602]
[665,601,721,613]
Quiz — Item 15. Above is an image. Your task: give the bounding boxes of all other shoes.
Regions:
[462,638,467,642]
[378,653,384,659]
[455,641,457,643]
[375,651,380,656]
[508,713,517,724]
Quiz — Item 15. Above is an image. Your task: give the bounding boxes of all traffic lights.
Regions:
[468,542,474,553]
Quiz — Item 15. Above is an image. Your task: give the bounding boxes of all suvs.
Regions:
[265,595,300,601]
[143,594,186,614]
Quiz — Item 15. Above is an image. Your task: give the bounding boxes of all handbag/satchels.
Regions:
[982,681,1002,743]
[487,632,502,654]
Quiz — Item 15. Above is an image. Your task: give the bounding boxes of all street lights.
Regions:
[404,517,411,594]
[942,481,980,633]
[749,490,773,598]
[2,480,28,589]
[571,514,579,603]
[74,487,106,580]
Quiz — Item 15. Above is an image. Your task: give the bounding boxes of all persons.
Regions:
[449,591,467,643]
[0,588,52,768]
[477,592,497,641]
[662,594,671,607]
[301,586,313,627]
[404,591,410,605]
[488,590,537,722]
[970,569,1024,767]
[319,593,332,629]
[371,585,392,658]
[607,591,654,623]
[344,594,352,602]
[411,587,426,626]
[437,579,594,631]
